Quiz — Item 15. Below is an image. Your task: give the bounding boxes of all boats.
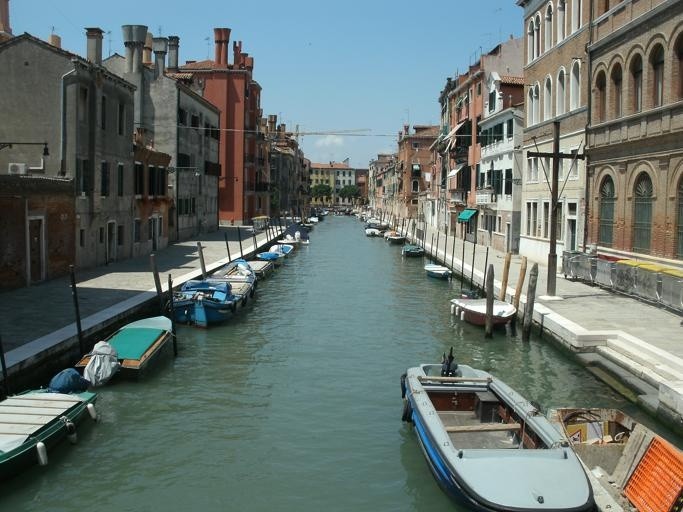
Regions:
[73,312,173,381]
[545,405,682,511]
[274,205,405,247]
[244,259,273,281]
[0,383,100,485]
[400,244,424,257]
[254,244,293,267]
[423,262,453,279]
[448,297,518,330]
[166,258,256,330]
[397,346,594,511]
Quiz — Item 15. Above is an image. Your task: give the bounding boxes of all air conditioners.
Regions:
[7,162,28,176]
